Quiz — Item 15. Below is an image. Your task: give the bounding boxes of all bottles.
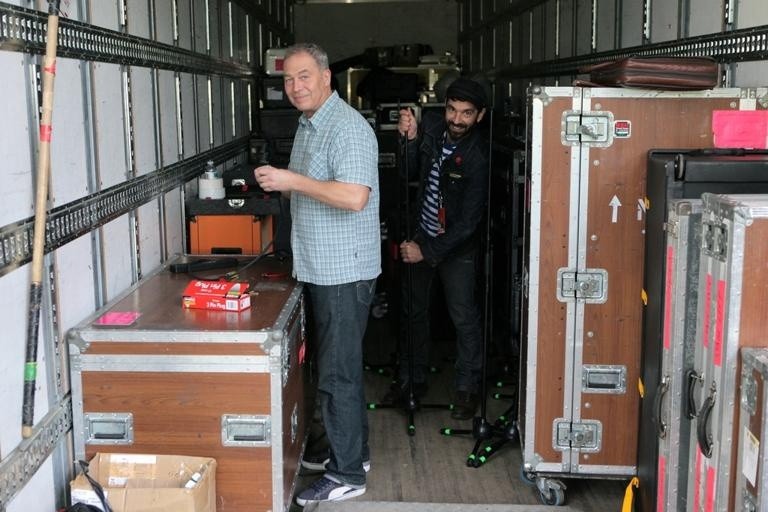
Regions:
[205,160,218,179]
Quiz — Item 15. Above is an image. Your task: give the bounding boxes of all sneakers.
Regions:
[296,473,365,507]
[301,445,370,473]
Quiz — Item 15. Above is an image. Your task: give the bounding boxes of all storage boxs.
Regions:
[70,453,216,512]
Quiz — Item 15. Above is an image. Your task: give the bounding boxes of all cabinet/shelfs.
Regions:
[517,84,768,512]
[67,255,312,512]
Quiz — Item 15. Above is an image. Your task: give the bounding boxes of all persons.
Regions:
[379,78,488,421]
[254,43,384,506]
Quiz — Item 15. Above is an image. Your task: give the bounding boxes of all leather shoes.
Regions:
[451,390,482,420]
[392,384,420,416]
